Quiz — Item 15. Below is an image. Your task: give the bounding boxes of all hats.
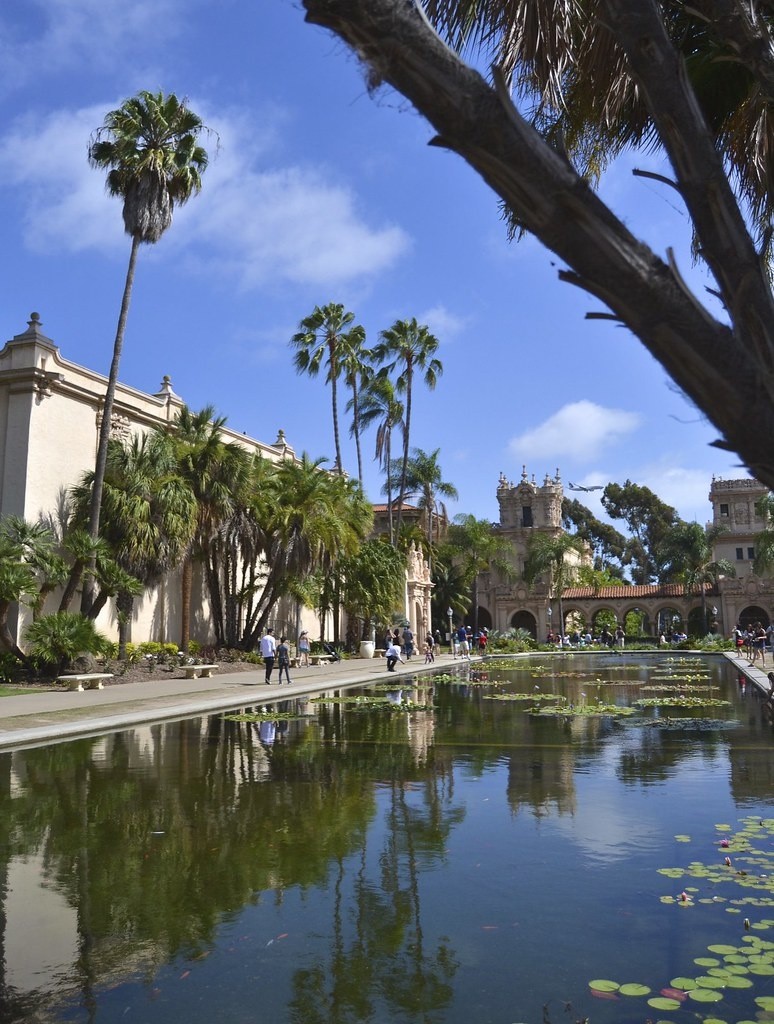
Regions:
[435,630,440,635]
[466,626,471,628]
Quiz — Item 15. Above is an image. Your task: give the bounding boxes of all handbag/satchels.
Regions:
[738,640,743,645]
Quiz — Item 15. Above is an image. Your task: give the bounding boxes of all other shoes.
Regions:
[266,678,271,684]
[735,656,740,658]
[469,659,471,660]
[298,664,301,668]
[745,657,749,660]
[388,667,397,672]
[278,682,283,685]
[289,680,294,685]
[462,657,464,659]
[763,665,765,668]
[455,657,457,660]
[748,663,754,667]
[307,666,310,668]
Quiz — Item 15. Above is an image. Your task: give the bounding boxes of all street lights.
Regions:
[447,606,454,655]
[546,605,553,633]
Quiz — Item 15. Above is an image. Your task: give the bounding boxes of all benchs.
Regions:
[374,648,388,657]
[56,673,114,692]
[308,654,334,665]
[178,664,220,680]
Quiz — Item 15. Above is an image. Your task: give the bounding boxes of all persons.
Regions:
[547,624,626,648]
[660,630,687,646]
[451,626,489,660]
[386,625,415,661]
[731,621,774,668]
[260,629,277,685]
[424,630,435,664]
[384,645,406,672]
[276,636,293,684]
[434,630,441,656]
[761,672,774,708]
[298,631,311,668]
